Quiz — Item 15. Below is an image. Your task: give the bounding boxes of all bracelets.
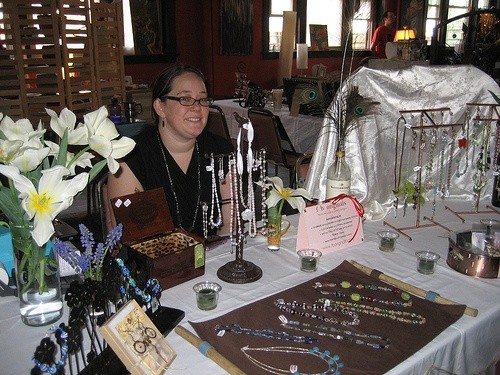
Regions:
[116,257,151,303]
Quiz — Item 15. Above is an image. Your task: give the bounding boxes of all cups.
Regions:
[192,281,222,310]
[296,249,322,272]
[415,251,441,274]
[377,230,400,252]
[272,89,283,112]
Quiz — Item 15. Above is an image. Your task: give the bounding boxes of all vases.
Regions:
[268,215,281,252]
[8,219,64,327]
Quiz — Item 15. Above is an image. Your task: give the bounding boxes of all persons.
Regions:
[371,10,395,58]
[107,64,239,255]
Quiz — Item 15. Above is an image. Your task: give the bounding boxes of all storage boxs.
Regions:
[110,186,207,294]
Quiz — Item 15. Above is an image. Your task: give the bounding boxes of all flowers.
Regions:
[0,104,137,295]
[256,176,314,232]
[53,223,122,281]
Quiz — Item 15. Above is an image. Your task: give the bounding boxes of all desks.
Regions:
[209,98,324,188]
[0,198,500,375]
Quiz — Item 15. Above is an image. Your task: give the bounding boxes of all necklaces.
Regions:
[209,149,266,253]
[157,130,201,231]
[314,281,412,307]
[319,298,425,324]
[274,299,359,326]
[240,347,343,375]
[213,325,317,344]
[284,321,390,349]
[393,106,500,218]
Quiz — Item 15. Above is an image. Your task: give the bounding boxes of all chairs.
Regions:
[205,104,311,189]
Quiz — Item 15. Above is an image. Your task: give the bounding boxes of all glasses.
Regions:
[160,96,214,107]
[385,15,397,19]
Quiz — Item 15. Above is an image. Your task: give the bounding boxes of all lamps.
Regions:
[394,26,415,61]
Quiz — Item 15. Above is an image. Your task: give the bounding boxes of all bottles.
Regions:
[124,94,142,124]
[110,98,122,124]
